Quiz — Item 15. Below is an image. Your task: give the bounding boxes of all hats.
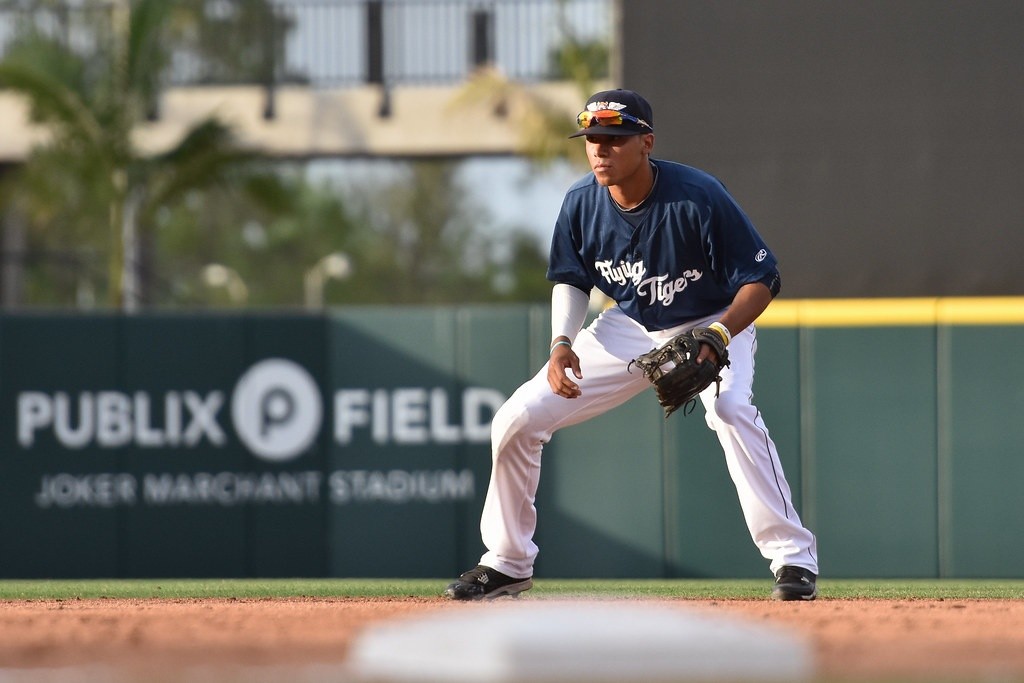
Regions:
[568,88,654,138]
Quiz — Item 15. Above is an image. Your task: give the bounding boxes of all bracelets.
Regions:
[549,341,571,355]
[708,322,731,346]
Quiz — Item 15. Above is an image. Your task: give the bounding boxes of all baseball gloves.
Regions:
[633,325,729,419]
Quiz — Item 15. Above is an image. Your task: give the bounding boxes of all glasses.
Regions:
[576,109,624,127]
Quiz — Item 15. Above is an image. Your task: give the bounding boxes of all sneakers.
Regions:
[770,565,817,600]
[443,565,532,601]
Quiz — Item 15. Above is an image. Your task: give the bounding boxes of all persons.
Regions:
[445,87,819,600]
